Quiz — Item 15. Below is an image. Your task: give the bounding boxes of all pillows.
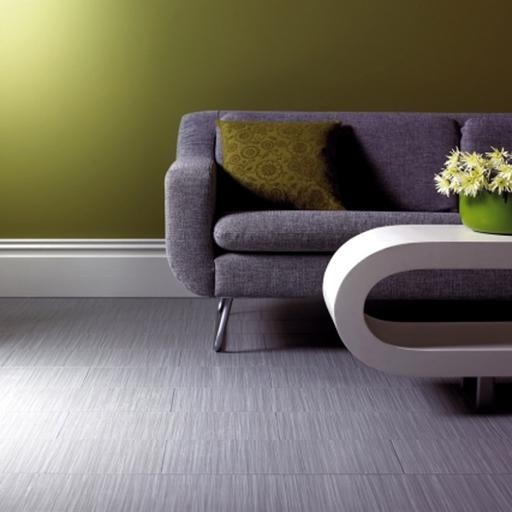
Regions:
[216,119,347,211]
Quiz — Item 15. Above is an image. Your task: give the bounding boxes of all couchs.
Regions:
[163,111,512,353]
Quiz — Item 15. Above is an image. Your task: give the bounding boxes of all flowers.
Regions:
[432,146,512,199]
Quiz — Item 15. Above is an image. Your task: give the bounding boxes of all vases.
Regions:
[458,189,512,236]
